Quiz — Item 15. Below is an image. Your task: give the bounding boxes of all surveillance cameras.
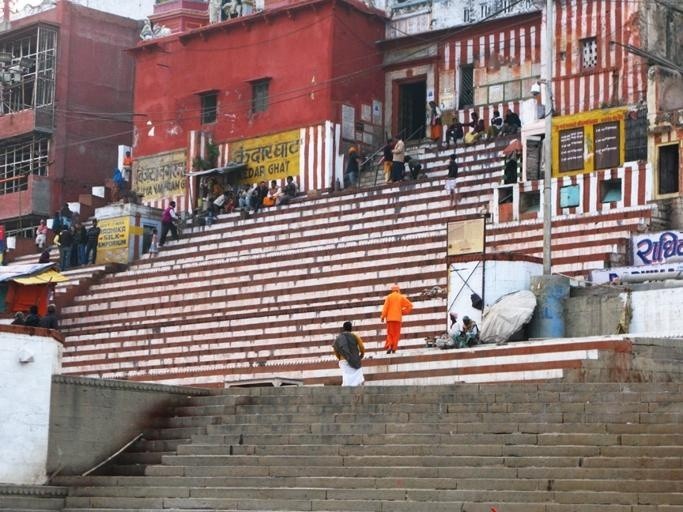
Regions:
[531,85,540,96]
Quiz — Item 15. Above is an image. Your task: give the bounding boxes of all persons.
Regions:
[111,151,132,202]
[380,285,413,354]
[332,322,365,386]
[148,176,296,258]
[436,311,480,349]
[345,101,521,210]
[10,203,101,330]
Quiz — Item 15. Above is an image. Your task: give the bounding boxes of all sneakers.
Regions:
[387,347,391,354]
